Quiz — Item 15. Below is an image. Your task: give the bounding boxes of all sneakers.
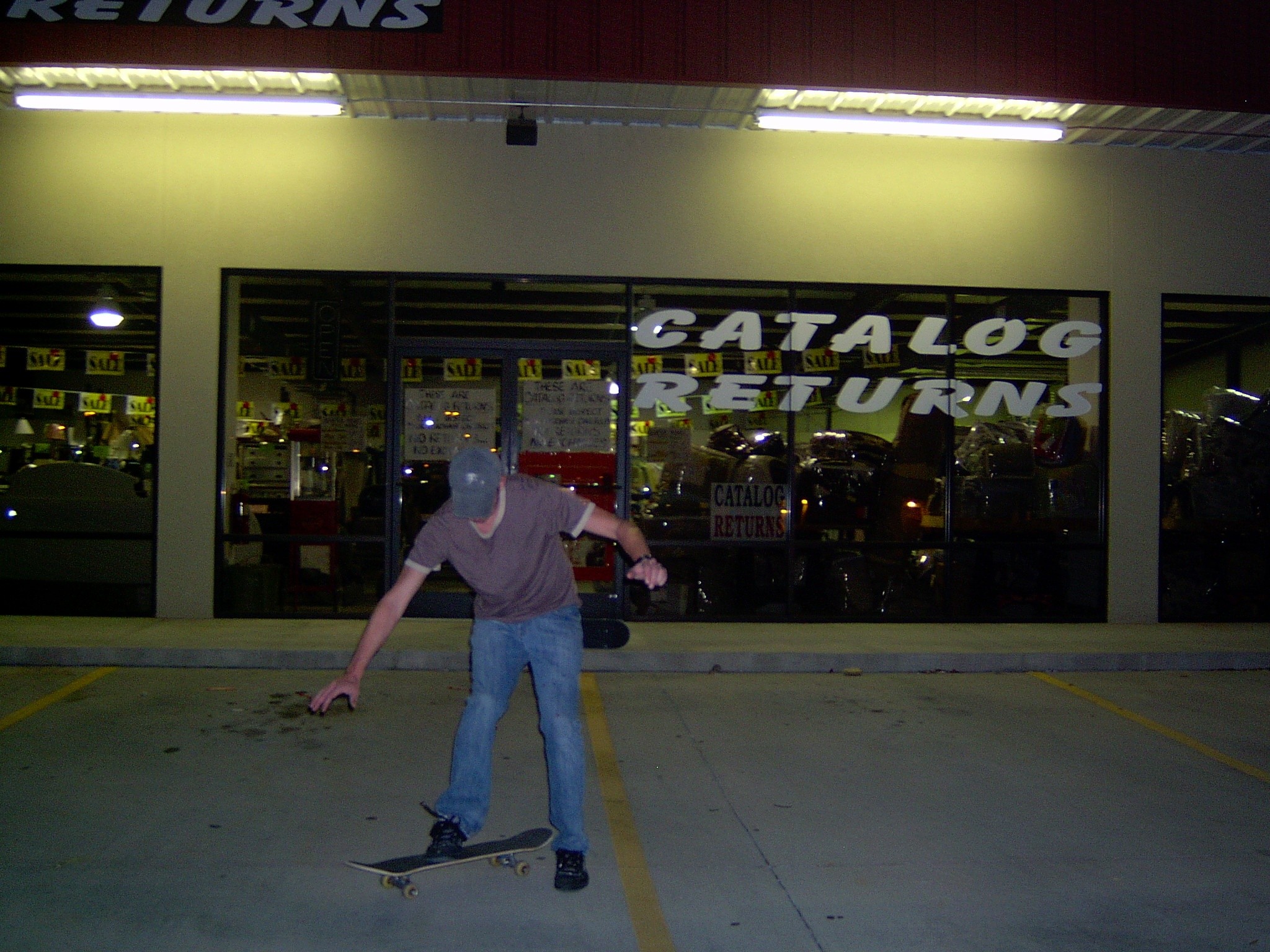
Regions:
[554,847,587,890]
[420,800,466,862]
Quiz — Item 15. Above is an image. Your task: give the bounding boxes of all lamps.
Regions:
[506,106,537,146]
[88,284,124,326]
[14,387,35,435]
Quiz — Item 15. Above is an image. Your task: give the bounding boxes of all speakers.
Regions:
[506,125,537,146]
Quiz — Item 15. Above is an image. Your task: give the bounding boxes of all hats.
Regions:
[448,446,499,520]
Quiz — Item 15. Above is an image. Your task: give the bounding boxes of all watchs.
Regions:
[635,553,653,564]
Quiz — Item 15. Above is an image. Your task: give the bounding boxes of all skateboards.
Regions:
[345,827,554,899]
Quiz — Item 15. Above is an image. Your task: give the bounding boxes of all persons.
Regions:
[309,446,668,891]
